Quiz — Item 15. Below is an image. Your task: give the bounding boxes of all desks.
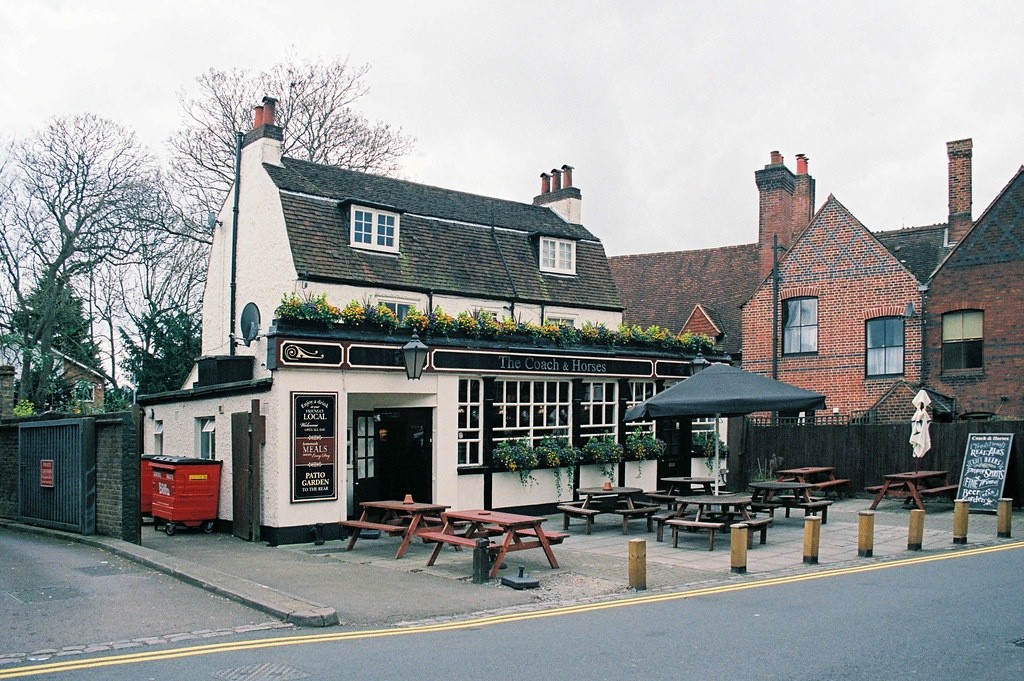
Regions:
[870,470,956,513]
[672,495,752,533]
[767,466,840,502]
[748,482,814,504]
[427,510,559,579]
[576,486,643,511]
[347,501,463,559]
[660,477,715,511]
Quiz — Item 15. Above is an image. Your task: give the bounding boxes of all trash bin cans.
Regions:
[141,454,169,526]
[148,456,223,536]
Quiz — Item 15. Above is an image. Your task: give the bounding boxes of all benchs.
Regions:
[557,477,851,551]
[339,516,570,556]
[864,483,904,491]
[919,484,959,494]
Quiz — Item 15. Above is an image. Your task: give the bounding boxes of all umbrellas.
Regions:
[908,390,931,474]
[623,364,826,498]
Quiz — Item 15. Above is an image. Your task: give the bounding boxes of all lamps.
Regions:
[401,323,430,380]
[681,347,711,376]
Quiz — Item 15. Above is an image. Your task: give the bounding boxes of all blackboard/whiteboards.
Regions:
[953,433,1015,511]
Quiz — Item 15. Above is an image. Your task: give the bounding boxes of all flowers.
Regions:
[493,427,729,501]
[273,290,716,348]
